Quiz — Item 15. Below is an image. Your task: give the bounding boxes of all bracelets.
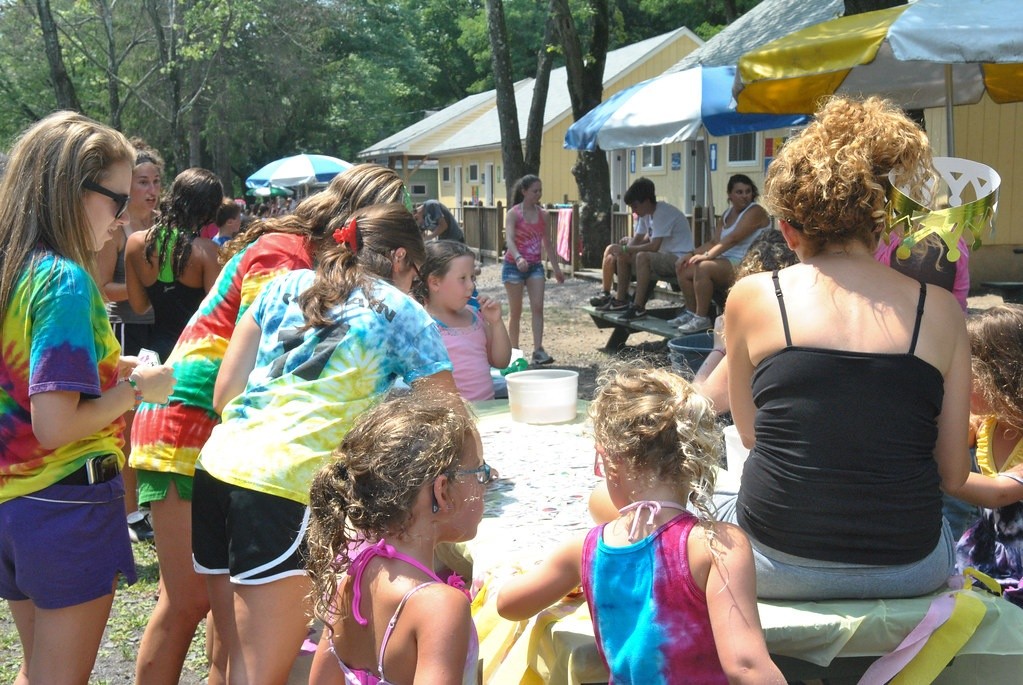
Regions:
[117,377,142,411]
[703,251,711,259]
[623,245,626,253]
[515,256,522,261]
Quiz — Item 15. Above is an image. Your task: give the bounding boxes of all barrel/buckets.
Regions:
[505,369,579,423]
[668,333,734,425]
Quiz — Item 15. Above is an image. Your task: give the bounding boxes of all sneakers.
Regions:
[619,306,647,322]
[127,513,156,544]
[666,312,694,327]
[596,299,629,310]
[589,293,612,306]
[679,315,713,334]
[531,348,554,364]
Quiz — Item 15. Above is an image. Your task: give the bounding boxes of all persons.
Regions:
[725,97,973,599]
[0,110,178,685]
[942,302,1023,610]
[501,175,564,364]
[309,391,491,685]
[590,173,772,333]
[92,136,512,414]
[191,201,482,685]
[497,369,789,685]
[128,164,414,685]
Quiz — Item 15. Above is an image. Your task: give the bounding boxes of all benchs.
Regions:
[582,299,688,340]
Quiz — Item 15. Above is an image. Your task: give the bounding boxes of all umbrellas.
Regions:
[564,0,1023,214]
[246,186,294,200]
[245,151,366,199]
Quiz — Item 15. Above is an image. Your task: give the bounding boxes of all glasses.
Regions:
[592,450,621,478]
[432,461,490,512]
[81,179,129,218]
[406,256,423,292]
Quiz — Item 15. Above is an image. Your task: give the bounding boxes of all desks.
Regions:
[605,272,727,350]
[436,398,1023,685]
